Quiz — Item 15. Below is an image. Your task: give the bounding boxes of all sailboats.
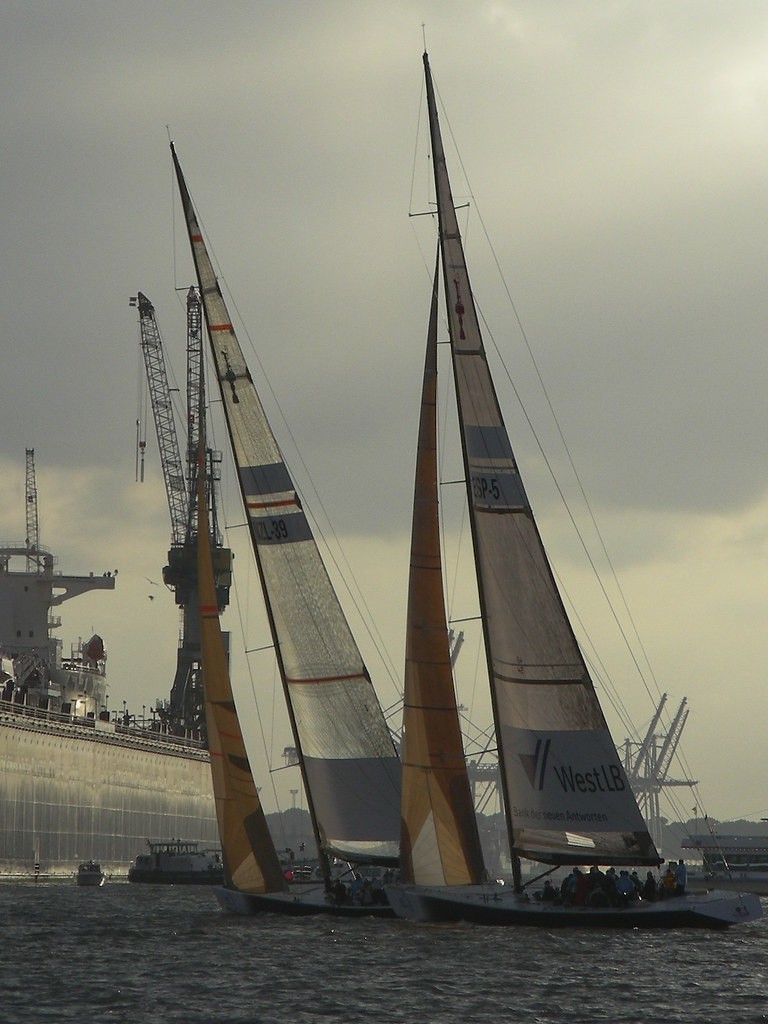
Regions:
[161,128,491,918]
[395,41,766,933]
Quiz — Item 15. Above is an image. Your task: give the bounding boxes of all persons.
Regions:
[542,858,688,910]
[331,867,406,907]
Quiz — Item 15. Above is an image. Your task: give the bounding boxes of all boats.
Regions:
[127,836,324,883]
[77,859,105,886]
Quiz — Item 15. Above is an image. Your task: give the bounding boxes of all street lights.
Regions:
[123,700,127,720]
[84,690,87,717]
[141,705,146,727]
[105,695,110,712]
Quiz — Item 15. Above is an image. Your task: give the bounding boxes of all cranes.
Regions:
[127,287,238,745]
[25,445,43,576]
[612,690,699,854]
[446,625,468,674]
